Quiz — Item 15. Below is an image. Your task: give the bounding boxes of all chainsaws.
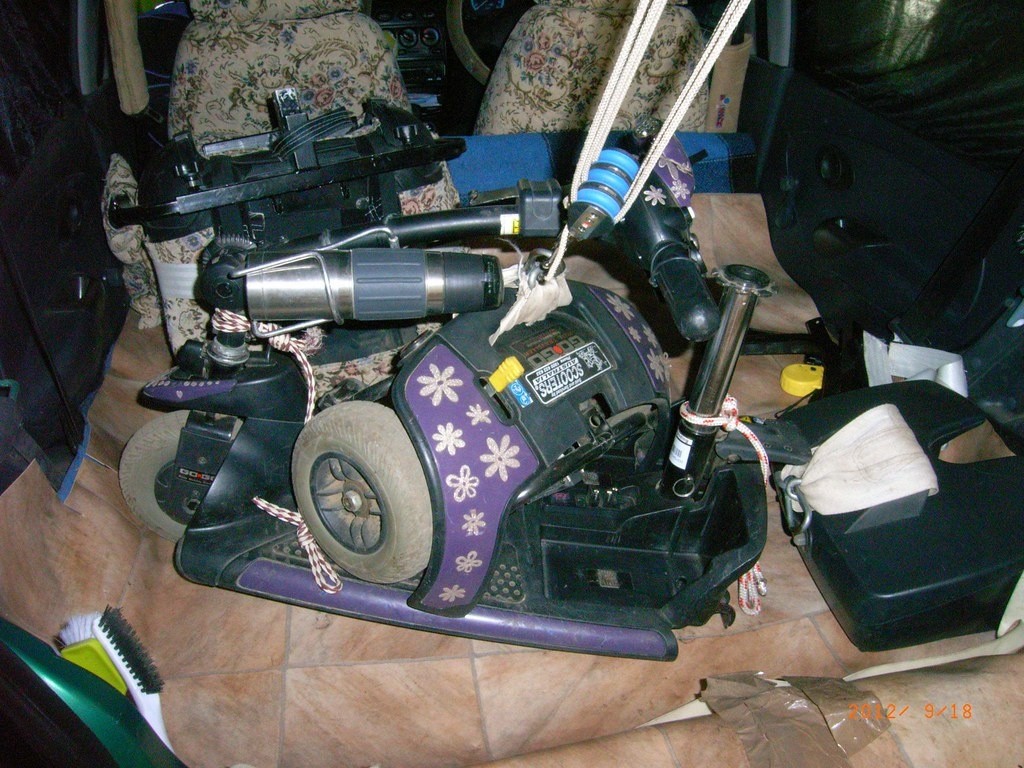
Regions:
[199,224,505,340]
[566,144,722,343]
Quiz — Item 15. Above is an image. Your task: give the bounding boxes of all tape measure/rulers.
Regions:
[778,361,825,397]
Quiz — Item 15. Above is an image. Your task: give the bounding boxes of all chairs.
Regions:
[168,0,420,159]
[473,0,711,134]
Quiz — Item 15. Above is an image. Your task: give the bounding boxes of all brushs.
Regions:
[58,611,128,700]
[93,602,174,756]
